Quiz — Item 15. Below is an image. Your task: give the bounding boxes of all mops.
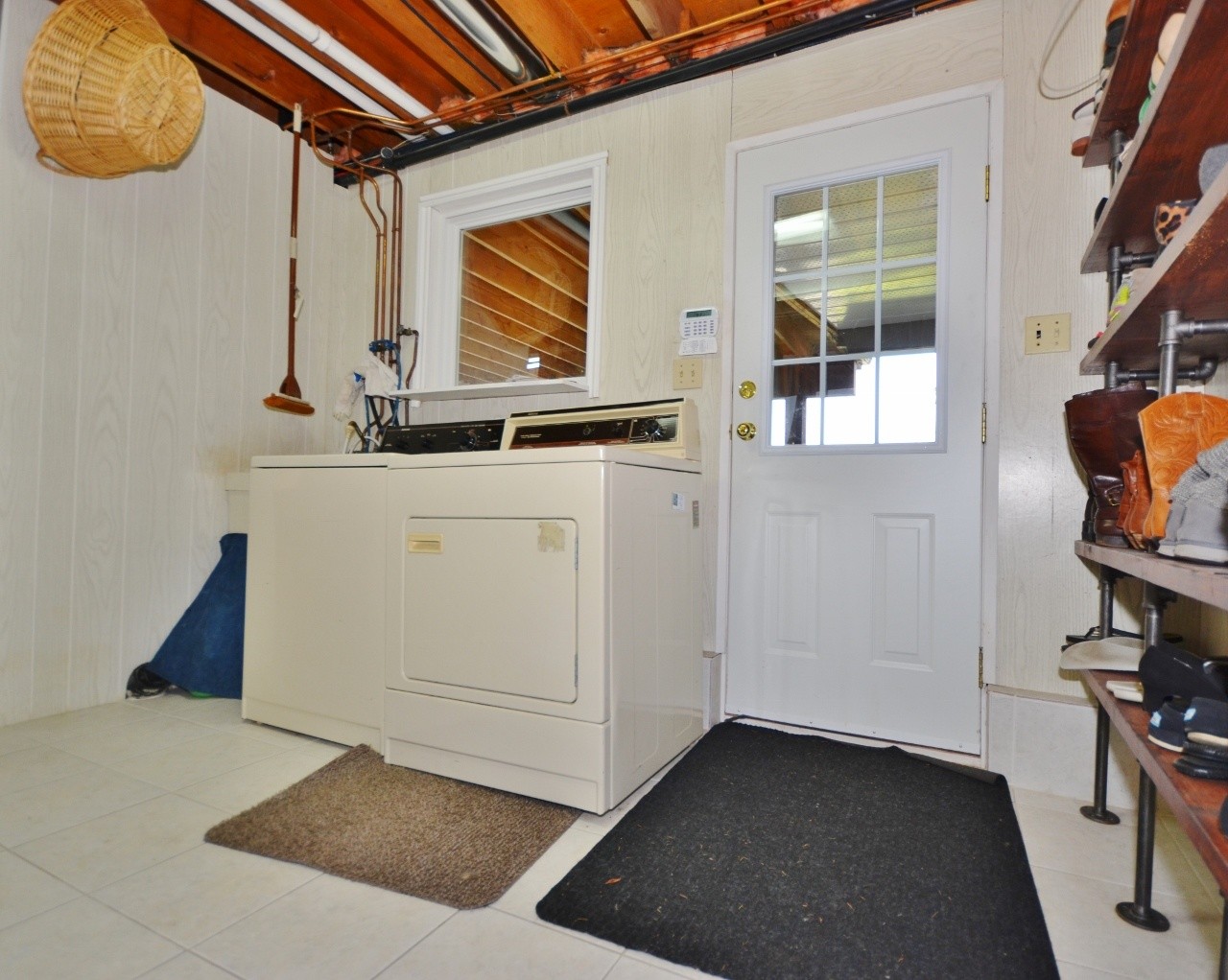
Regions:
[262,103,317,417]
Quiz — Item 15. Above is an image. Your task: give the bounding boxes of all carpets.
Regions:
[203,743,585,909]
[533,720,1061,979]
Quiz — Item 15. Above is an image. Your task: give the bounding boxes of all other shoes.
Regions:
[1071,2,1198,245]
[1147,696,1228,780]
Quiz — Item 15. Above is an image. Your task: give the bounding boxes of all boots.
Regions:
[1064,380,1228,564]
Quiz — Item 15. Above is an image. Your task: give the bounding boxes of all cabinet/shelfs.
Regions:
[1079,0,1228,980]
[247,468,715,816]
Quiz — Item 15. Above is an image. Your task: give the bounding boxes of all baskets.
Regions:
[23,0,205,180]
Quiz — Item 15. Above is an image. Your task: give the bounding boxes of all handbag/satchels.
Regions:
[1138,644,1228,716]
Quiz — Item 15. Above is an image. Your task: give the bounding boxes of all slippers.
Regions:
[1062,625,1183,653]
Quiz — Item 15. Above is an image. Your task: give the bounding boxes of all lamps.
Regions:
[206,1,568,150]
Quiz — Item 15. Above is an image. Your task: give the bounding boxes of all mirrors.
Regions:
[421,151,609,387]
